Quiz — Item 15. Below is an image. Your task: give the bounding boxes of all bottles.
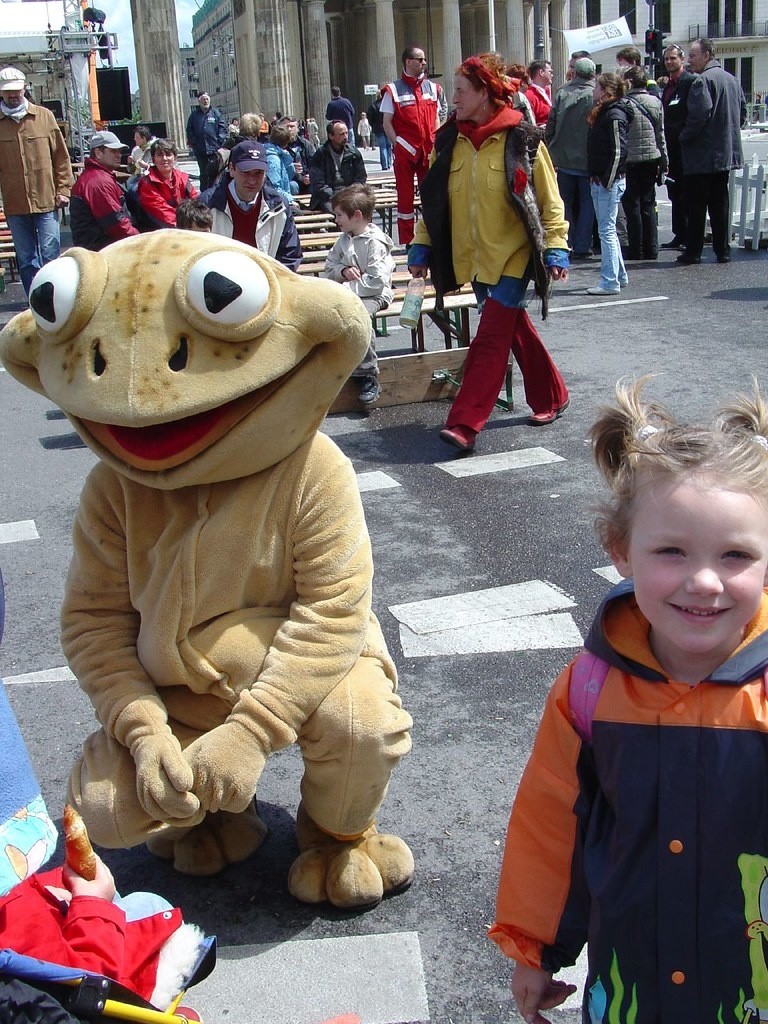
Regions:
[399,270,426,329]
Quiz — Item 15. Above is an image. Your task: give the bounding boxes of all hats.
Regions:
[230,140,268,172]
[91,131,130,150]
[0,68,26,91]
[197,91,209,98]
[576,58,597,77]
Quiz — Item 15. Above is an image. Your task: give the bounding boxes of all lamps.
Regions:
[83,7,106,23]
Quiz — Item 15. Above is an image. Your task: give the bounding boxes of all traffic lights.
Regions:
[645,30,658,53]
[656,30,667,59]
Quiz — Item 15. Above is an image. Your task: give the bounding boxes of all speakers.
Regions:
[44,99,67,142]
[95,67,133,121]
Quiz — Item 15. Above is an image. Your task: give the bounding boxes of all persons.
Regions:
[176,200,213,233]
[408,52,576,453]
[584,73,632,299]
[379,47,448,248]
[508,40,744,263]
[69,131,139,252]
[488,370,768,1024]
[196,140,304,275]
[186,86,379,215]
[0,847,175,1002]
[323,185,396,409]
[136,138,200,230]
[122,126,160,194]
[0,67,72,304]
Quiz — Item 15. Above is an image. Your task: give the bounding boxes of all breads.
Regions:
[62,804,96,880]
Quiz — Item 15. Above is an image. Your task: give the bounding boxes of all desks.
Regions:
[71,162,131,188]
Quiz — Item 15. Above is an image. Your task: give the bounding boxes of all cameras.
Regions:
[657,172,677,187]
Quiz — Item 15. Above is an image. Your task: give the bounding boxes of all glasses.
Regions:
[408,57,427,63]
[543,69,554,75]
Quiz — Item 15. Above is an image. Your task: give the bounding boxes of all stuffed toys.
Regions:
[0,229,421,913]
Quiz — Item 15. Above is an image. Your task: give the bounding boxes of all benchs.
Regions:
[0,200,19,293]
[290,174,513,414]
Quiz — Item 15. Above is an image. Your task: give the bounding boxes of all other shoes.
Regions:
[717,252,730,263]
[382,168,387,170]
[702,233,714,242]
[622,246,641,259]
[677,253,700,264]
[662,237,683,248]
[573,250,594,259]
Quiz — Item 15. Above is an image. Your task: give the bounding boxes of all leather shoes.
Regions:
[440,425,476,450]
[533,397,570,422]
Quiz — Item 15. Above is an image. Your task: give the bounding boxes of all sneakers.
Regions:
[358,376,381,405]
[586,285,616,294]
